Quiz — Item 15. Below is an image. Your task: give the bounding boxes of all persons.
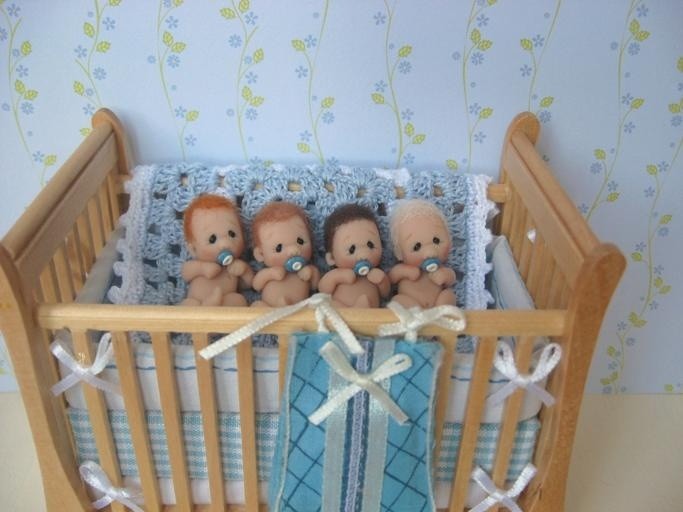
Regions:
[318,202,391,310]
[174,194,253,307]
[386,200,457,310]
[248,201,320,310]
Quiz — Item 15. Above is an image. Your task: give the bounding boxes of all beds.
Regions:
[0,107,626,512]
